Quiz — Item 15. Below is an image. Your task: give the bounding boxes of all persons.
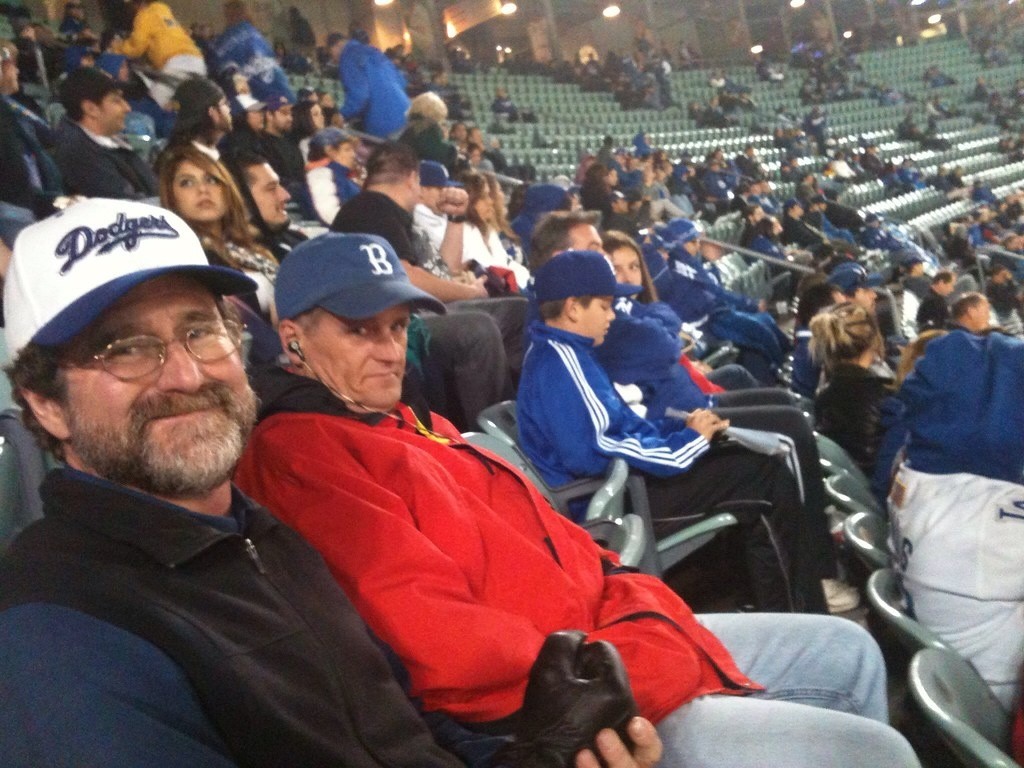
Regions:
[0,1,1022,768]
[234,233,926,768]
[1,196,667,767]
[515,249,835,616]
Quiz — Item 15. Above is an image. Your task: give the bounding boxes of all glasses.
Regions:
[53,319,248,383]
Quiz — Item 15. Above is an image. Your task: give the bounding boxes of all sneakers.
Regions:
[821,577,860,612]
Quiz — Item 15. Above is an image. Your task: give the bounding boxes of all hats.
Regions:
[234,92,266,112]
[666,219,700,244]
[2,197,260,369]
[898,248,927,262]
[420,159,465,186]
[254,83,296,112]
[553,174,582,194]
[274,232,447,322]
[535,250,643,303]
[311,126,349,146]
[172,78,222,131]
[825,262,883,290]
[60,66,128,106]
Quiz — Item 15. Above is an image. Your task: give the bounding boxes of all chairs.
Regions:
[0,37,1024,768]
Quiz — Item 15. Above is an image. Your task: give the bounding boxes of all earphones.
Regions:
[287,340,306,362]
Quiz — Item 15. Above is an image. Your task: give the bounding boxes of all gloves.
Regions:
[478,629,633,768]
